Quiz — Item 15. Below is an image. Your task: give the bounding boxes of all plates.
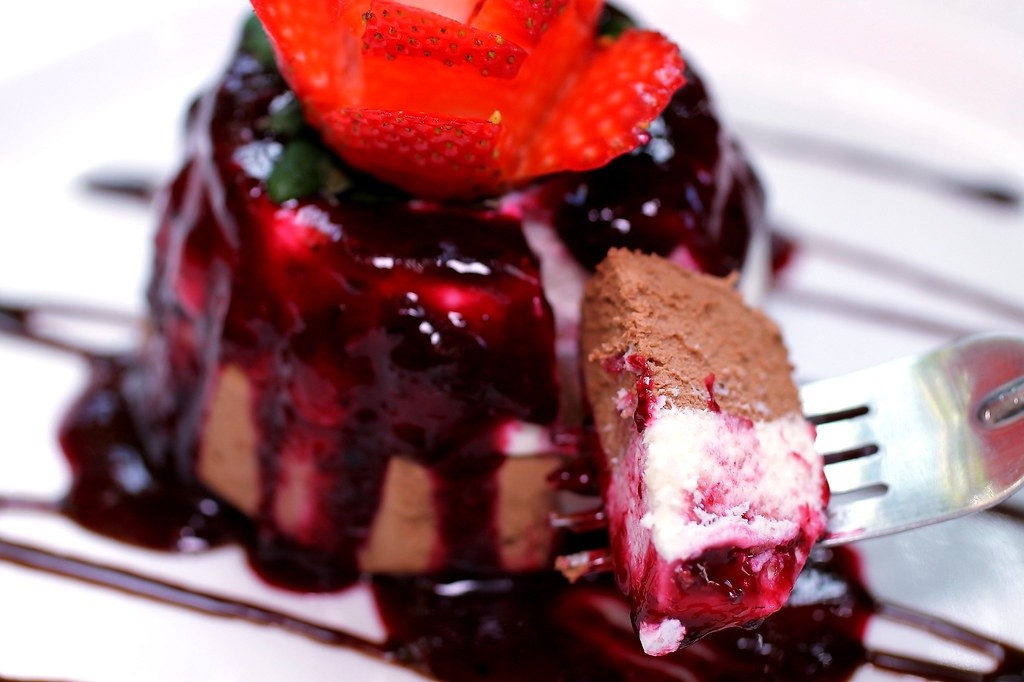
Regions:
[0,0,1024,682]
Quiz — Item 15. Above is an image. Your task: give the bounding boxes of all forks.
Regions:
[796,334,1024,549]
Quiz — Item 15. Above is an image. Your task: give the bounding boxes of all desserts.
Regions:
[52,0,880,682]
